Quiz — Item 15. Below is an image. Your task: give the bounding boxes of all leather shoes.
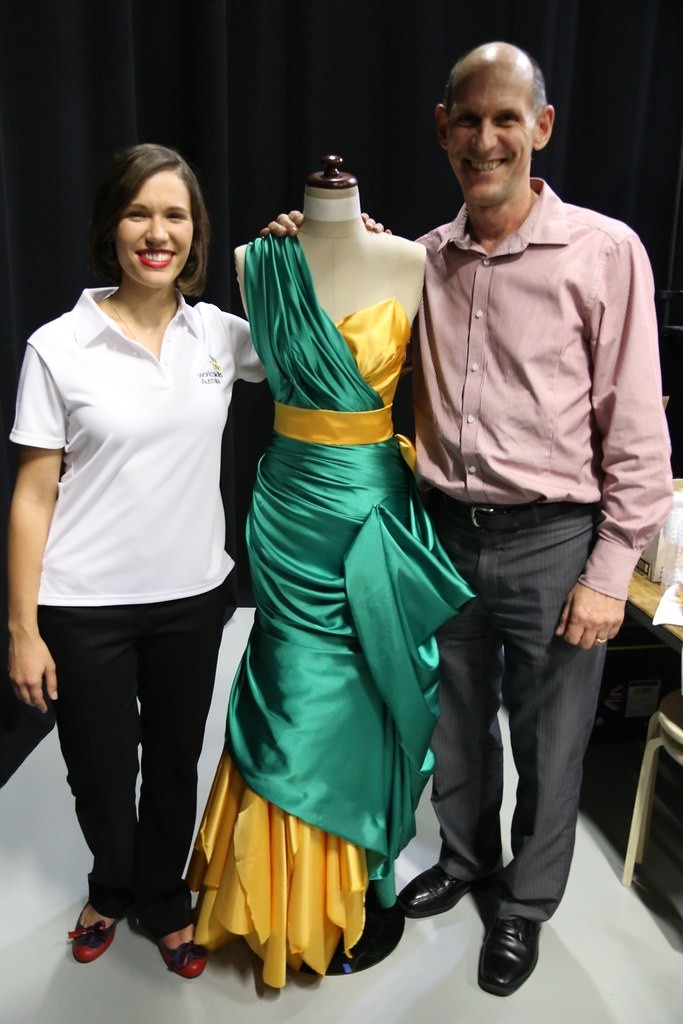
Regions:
[158,936,207,977]
[478,918,540,997]
[68,901,119,963]
[397,862,506,918]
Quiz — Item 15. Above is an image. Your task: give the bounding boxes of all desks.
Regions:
[606,568,683,670]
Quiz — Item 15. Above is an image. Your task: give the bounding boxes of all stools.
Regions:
[622,689,683,887]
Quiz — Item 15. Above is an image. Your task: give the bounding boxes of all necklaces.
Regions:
[108,294,144,350]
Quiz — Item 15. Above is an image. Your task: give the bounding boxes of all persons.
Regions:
[7,143,391,979]
[261,40,675,997]
[184,154,476,990]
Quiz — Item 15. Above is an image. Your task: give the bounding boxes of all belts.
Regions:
[434,490,578,529]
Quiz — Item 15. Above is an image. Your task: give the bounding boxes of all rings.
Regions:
[595,638,607,646]
